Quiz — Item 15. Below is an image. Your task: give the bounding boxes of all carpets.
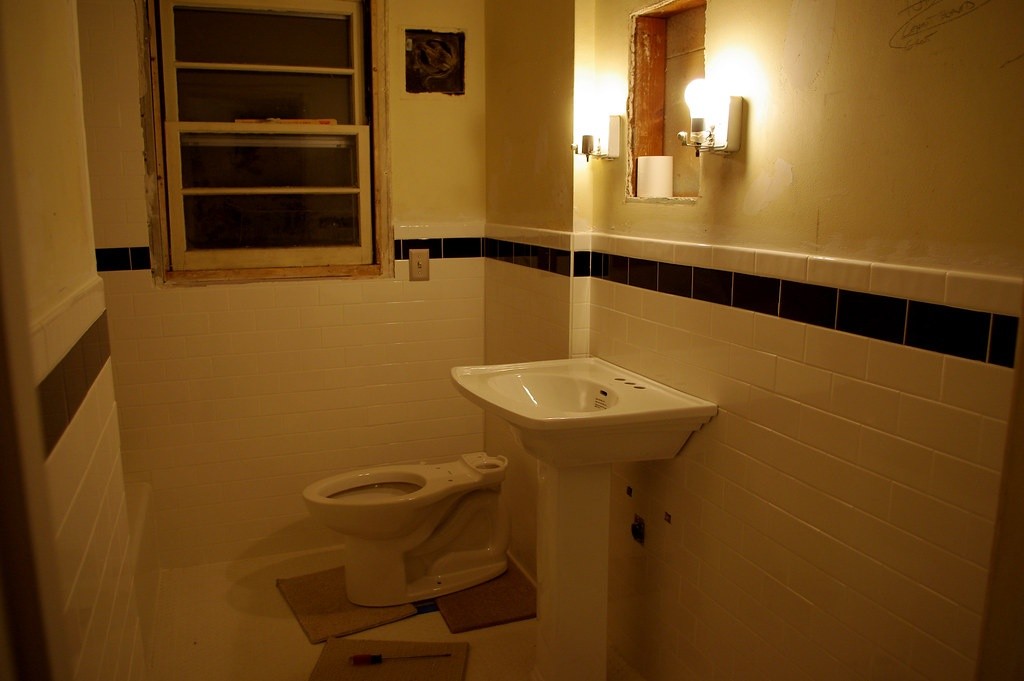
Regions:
[434,554,538,634]
[276,564,419,645]
[307,637,473,681]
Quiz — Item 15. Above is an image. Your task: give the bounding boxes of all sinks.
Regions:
[451,358,718,463]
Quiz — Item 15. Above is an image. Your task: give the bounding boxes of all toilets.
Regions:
[304,452,507,607]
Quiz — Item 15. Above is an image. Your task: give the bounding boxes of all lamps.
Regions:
[676,77,744,156]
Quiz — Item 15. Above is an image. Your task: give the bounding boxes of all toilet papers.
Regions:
[635,155,674,198]
